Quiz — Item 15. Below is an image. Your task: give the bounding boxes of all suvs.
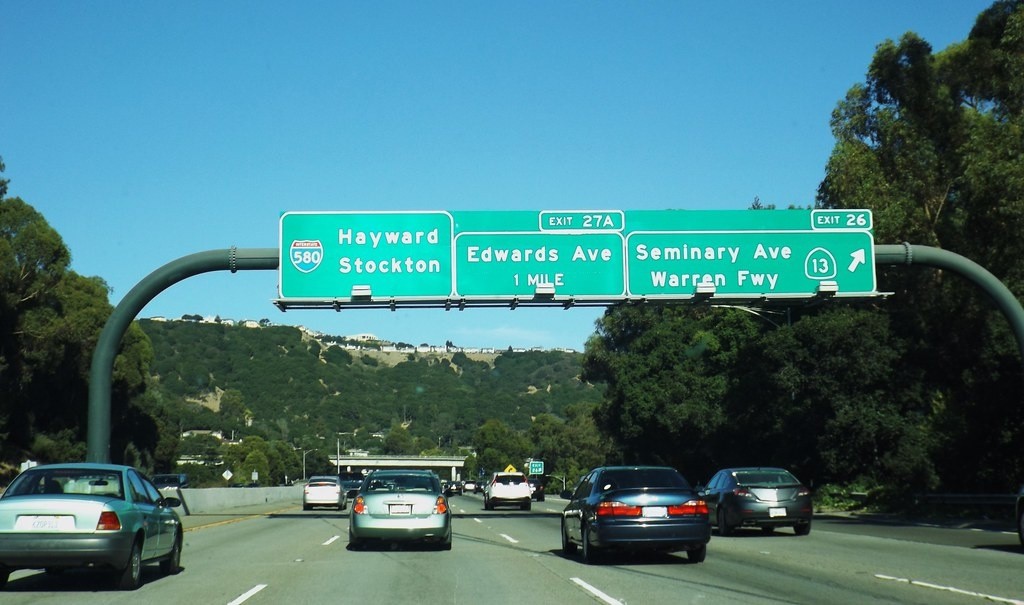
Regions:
[481,471,533,511]
[337,471,396,498]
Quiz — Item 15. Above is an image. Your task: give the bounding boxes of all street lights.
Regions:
[545,473,565,491]
[303,448,318,481]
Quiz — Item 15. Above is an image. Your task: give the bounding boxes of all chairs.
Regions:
[44,481,63,494]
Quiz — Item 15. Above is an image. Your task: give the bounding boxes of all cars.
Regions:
[347,468,457,551]
[150,472,189,492]
[438,478,477,498]
[0,462,185,591]
[473,481,488,494]
[302,475,349,511]
[560,465,712,565]
[526,478,546,502]
[695,467,813,537]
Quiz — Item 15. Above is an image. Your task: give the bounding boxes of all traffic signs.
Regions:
[624,229,877,298]
[278,210,454,299]
[451,232,628,300]
